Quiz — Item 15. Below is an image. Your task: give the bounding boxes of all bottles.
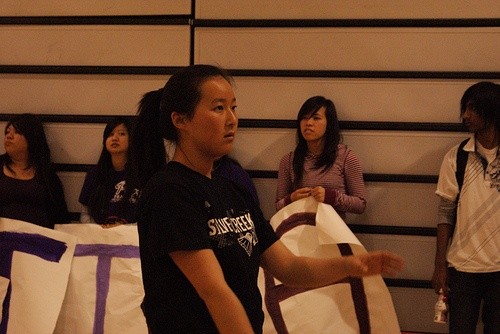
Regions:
[433,285,449,325]
